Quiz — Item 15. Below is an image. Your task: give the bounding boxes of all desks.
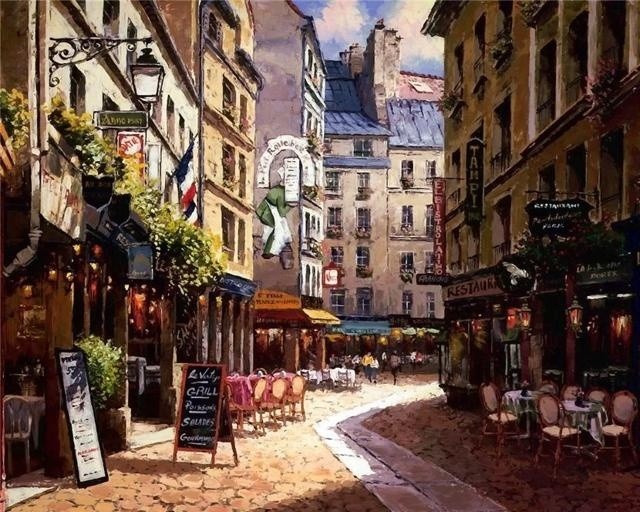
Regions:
[5,394,44,448]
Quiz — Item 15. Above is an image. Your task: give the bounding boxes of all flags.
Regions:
[175,143,198,227]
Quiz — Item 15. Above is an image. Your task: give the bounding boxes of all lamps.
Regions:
[48,253,60,287]
[566,295,584,338]
[515,299,534,341]
[21,278,35,299]
[46,34,172,103]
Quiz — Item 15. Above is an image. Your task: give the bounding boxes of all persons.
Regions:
[328,350,423,386]
[255,166,298,260]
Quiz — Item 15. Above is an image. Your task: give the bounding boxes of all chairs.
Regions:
[226,368,313,433]
[130,354,162,423]
[299,368,356,389]
[477,380,638,477]
[1,393,31,473]
